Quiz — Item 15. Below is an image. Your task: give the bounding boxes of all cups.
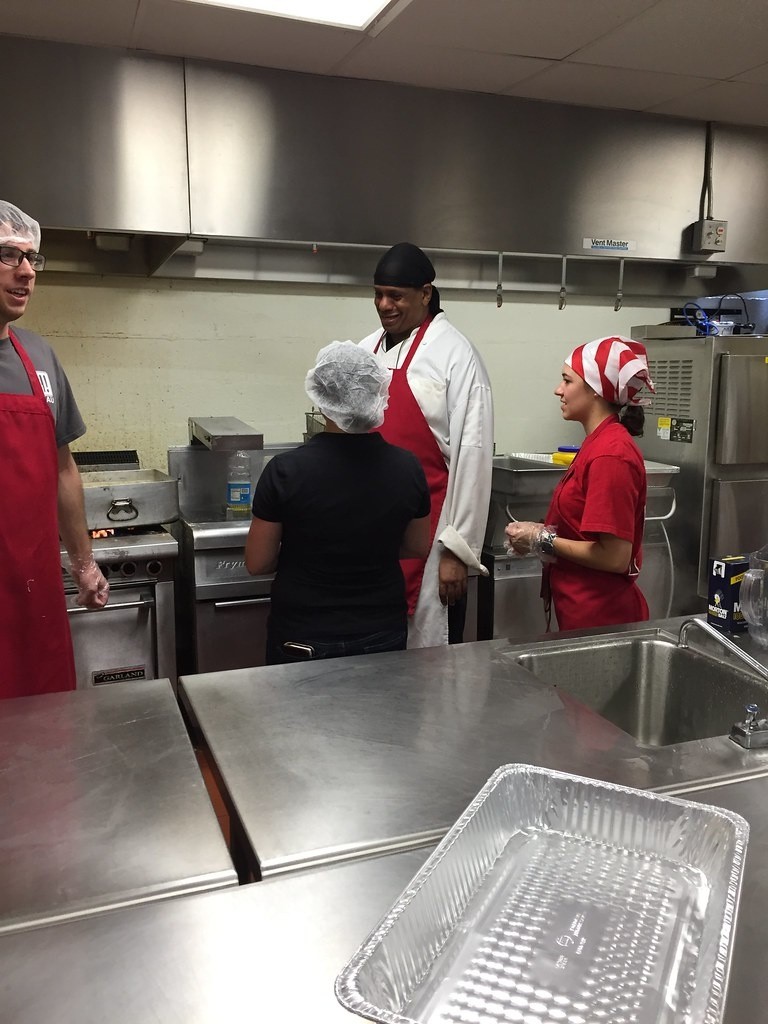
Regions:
[739,544,768,648]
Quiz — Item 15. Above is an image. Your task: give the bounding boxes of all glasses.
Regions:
[0,246,46,271]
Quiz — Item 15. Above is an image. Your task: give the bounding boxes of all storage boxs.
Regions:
[491,456,568,497]
[707,553,752,633]
[78,469,180,531]
[334,763,751,1024]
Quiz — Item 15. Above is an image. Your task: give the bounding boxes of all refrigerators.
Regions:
[629,319,768,614]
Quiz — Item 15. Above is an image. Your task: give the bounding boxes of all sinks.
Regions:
[493,627,768,748]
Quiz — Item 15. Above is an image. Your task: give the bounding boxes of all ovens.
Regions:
[54,541,179,701]
[182,520,276,674]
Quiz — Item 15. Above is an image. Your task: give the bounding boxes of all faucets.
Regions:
[677,617,768,750]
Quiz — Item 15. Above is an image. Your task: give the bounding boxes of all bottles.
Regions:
[552,445,580,467]
[226,450,252,511]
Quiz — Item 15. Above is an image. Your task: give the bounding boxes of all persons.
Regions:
[0,202,109,700]
[354,240,495,648]
[506,335,652,637]
[244,342,430,665]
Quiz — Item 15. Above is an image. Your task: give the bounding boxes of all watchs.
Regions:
[540,533,557,557]
